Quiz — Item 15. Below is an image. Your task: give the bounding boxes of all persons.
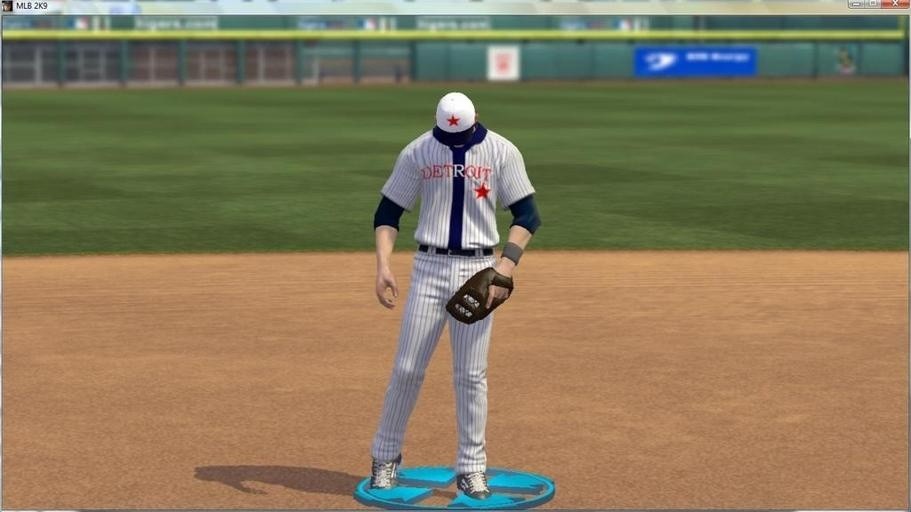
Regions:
[366,90,543,503]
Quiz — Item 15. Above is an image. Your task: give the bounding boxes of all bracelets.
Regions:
[501,240,525,266]
